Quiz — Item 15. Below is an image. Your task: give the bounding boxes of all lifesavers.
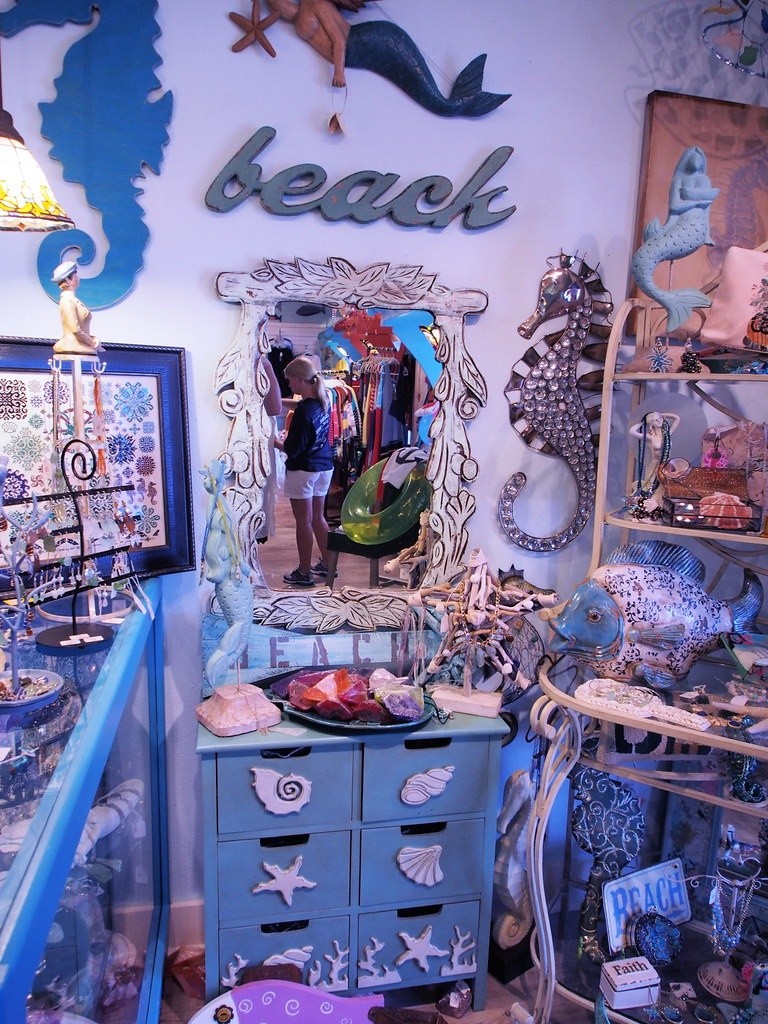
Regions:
[340,457,432,545]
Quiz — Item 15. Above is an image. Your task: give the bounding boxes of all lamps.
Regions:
[0,54,75,233]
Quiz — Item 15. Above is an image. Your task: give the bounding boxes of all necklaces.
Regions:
[711,876,756,957]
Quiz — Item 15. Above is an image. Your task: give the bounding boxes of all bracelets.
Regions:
[659,1004,684,1024]
[695,1005,719,1024]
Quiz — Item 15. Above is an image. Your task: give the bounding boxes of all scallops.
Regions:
[397,846,444,887]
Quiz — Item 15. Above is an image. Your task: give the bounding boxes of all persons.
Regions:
[258,351,283,543]
[669,146,716,246]
[628,412,680,507]
[275,357,338,585]
[52,262,106,355]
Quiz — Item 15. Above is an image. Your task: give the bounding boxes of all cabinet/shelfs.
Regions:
[0,574,170,1024]
[195,613,510,1013]
[527,243,767,1023]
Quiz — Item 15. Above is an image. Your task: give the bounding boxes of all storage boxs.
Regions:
[598,956,661,1010]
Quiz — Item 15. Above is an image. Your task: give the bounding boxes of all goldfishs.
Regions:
[548,541,768,686]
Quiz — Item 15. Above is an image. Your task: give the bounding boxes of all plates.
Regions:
[267,689,436,730]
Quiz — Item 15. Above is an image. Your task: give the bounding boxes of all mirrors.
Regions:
[209,256,489,632]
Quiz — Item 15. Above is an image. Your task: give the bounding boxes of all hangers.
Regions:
[357,346,408,376]
[303,345,313,356]
[272,336,287,349]
[314,369,346,389]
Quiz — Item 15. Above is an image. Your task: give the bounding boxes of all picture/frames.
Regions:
[0,336,197,602]
